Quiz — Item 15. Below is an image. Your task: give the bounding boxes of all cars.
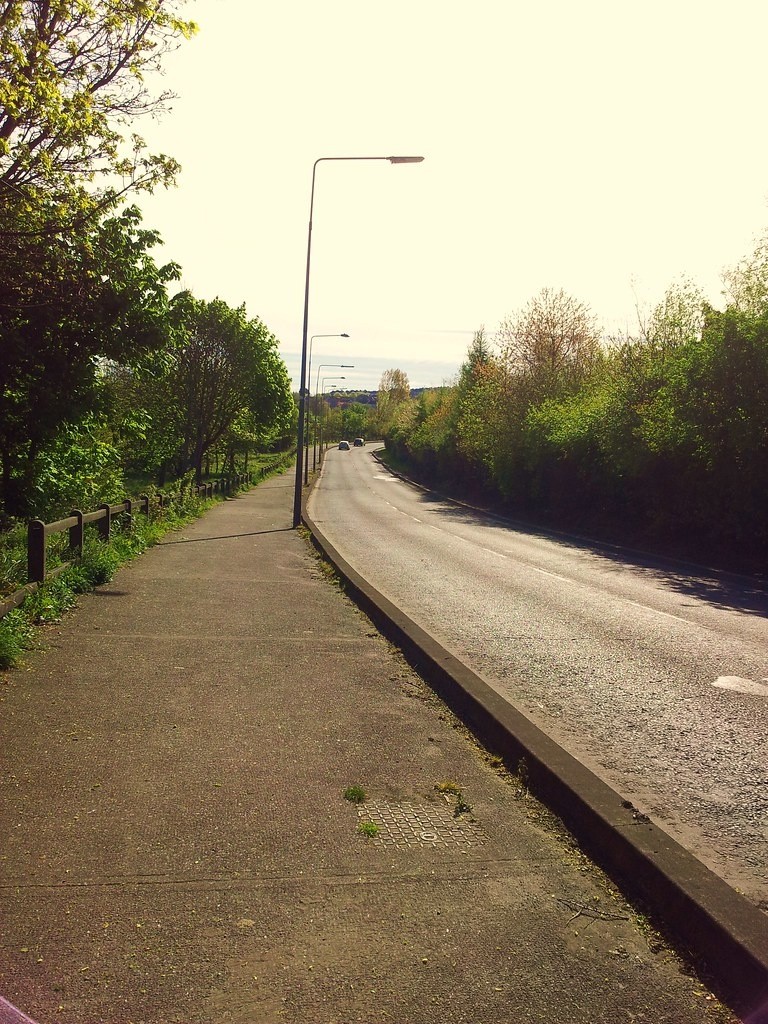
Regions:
[339,441,350,450]
[354,438,366,446]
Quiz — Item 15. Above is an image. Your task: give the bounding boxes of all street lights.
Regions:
[324,385,336,448]
[292,155,426,528]
[304,333,350,485]
[313,365,354,472]
[319,377,346,464]
